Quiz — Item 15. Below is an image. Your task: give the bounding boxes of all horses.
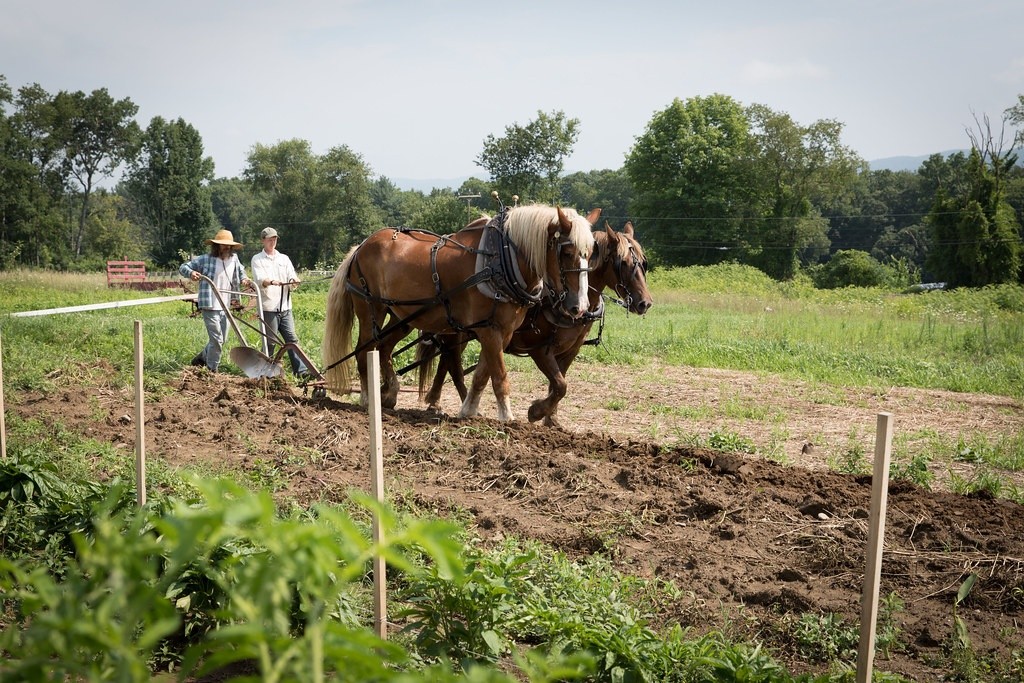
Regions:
[321,202,601,423]
[411,220,653,432]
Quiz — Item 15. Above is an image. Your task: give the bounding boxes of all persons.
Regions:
[180,229,250,375]
[251,227,316,380]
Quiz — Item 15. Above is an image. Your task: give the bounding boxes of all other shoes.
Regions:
[191,355,216,373]
[294,372,315,380]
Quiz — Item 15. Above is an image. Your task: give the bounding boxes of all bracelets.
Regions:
[271,279,275,285]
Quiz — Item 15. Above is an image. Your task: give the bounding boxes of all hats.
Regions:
[261,227,280,239]
[205,230,243,249]
